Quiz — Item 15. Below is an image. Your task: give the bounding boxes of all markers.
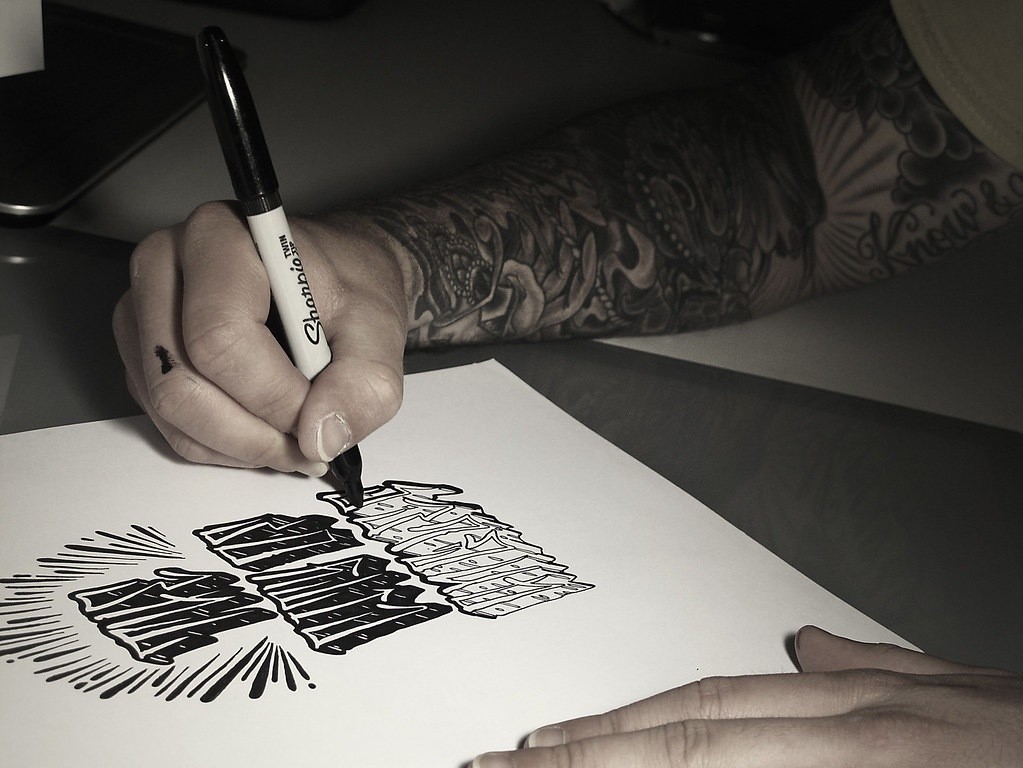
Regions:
[197,22,368,508]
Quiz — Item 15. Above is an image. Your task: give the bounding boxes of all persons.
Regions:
[112,0,1022,767]
